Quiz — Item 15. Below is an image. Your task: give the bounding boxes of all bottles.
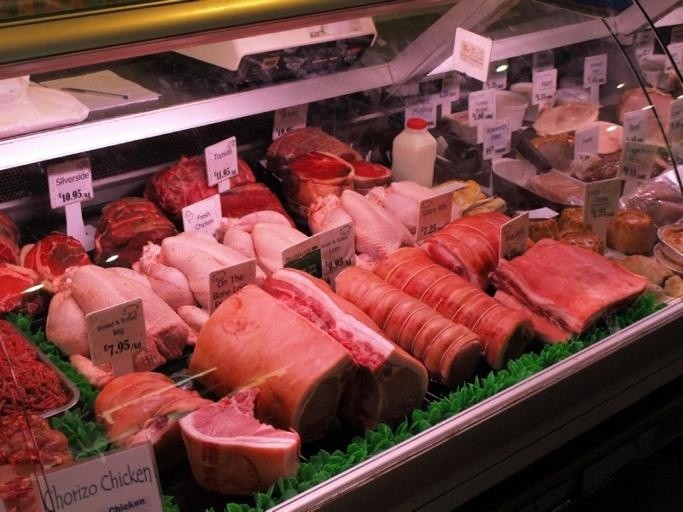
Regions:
[393,118,438,187]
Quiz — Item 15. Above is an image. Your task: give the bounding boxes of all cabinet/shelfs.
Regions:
[1,2,683,511]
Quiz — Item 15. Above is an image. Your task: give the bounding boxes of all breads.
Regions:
[450,180,506,216]
[521,85,683,297]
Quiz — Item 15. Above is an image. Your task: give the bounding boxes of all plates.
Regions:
[657,224,683,266]
[653,242,683,277]
